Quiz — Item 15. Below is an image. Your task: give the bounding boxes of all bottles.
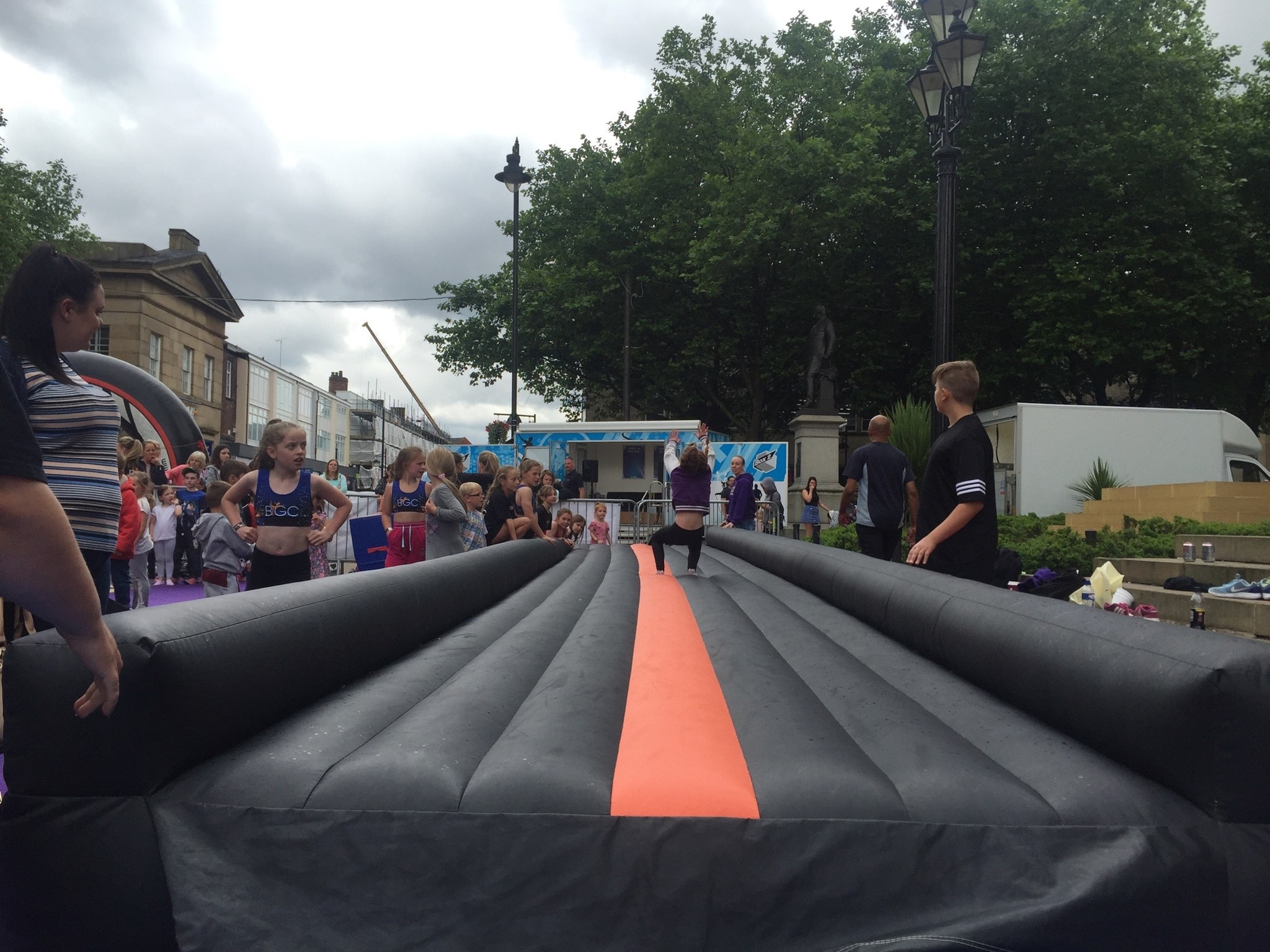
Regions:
[1081,580,1095,608]
[1189,587,1206,631]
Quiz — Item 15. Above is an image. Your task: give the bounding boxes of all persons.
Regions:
[381,447,432,568]
[0,243,255,718]
[838,415,920,564]
[450,450,587,547]
[309,494,333,580]
[375,462,396,514]
[318,458,347,494]
[422,447,468,562]
[717,456,785,537]
[221,418,352,593]
[906,360,998,587]
[798,476,831,541]
[587,501,612,545]
[651,423,712,576]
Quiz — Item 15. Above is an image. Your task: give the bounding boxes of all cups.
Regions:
[1110,588,1134,608]
[1007,580,1020,592]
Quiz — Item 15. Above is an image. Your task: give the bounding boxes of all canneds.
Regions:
[1202,542,1215,562]
[1183,542,1196,562]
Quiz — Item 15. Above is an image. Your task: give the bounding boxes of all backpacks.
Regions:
[770,491,785,530]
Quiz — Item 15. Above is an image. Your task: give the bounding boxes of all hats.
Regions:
[182,466,200,476]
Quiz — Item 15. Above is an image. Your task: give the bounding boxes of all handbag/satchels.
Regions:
[991,546,1023,588]
[1069,561,1124,609]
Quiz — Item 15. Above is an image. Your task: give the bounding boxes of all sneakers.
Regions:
[1258,578,1270,600]
[1208,574,1262,599]
[1103,602,1133,617]
[1134,606,1159,622]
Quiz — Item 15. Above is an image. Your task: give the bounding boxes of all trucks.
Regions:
[970,402,1270,515]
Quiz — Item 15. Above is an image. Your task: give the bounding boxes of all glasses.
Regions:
[469,491,486,498]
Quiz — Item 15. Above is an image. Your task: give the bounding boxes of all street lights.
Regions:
[903,0,993,445]
[493,136,532,442]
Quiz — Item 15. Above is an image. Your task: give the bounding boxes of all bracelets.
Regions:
[233,522,245,530]
[385,527,393,535]
